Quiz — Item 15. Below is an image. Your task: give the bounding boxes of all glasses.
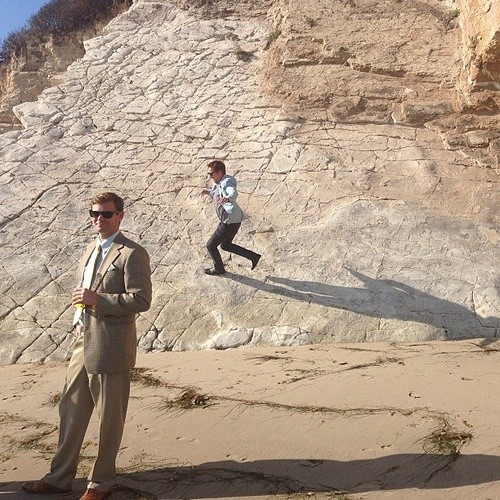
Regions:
[206,172,216,176]
[89,209,119,219]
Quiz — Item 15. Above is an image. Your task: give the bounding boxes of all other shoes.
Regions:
[250,254,261,270]
[203,267,225,275]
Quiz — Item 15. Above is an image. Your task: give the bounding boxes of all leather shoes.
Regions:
[79,488,112,500]
[22,479,67,493]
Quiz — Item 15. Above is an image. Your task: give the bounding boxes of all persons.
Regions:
[23,192,152,500]
[198,161,262,275]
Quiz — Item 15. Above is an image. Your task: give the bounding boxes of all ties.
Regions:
[73,243,102,327]
